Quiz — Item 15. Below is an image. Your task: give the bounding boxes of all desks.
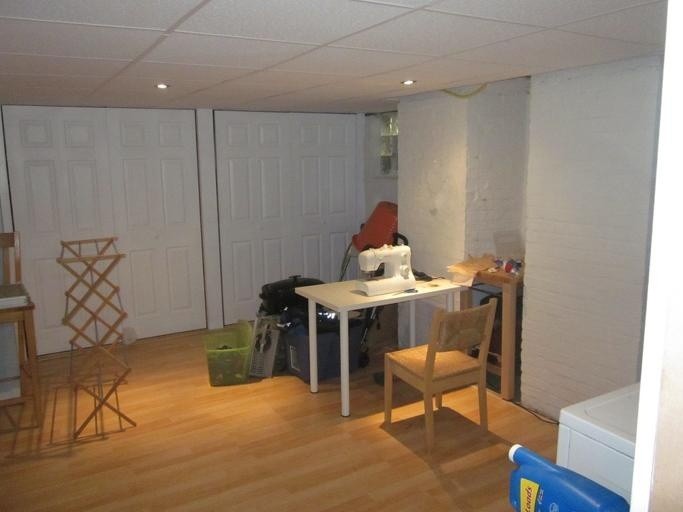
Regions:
[295,272,466,417]
[448,254,524,402]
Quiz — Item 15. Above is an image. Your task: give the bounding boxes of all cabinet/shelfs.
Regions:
[293,113,366,292]
[214,110,295,326]
[103,106,207,343]
[2,104,131,356]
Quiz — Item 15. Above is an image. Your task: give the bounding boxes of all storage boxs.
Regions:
[288,324,363,381]
[203,329,250,386]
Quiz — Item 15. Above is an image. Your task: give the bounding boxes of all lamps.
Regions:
[376,113,400,159]
[399,70,422,98]
[150,78,172,101]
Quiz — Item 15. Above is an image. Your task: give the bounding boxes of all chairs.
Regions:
[382,296,498,447]
[0,232,43,426]
